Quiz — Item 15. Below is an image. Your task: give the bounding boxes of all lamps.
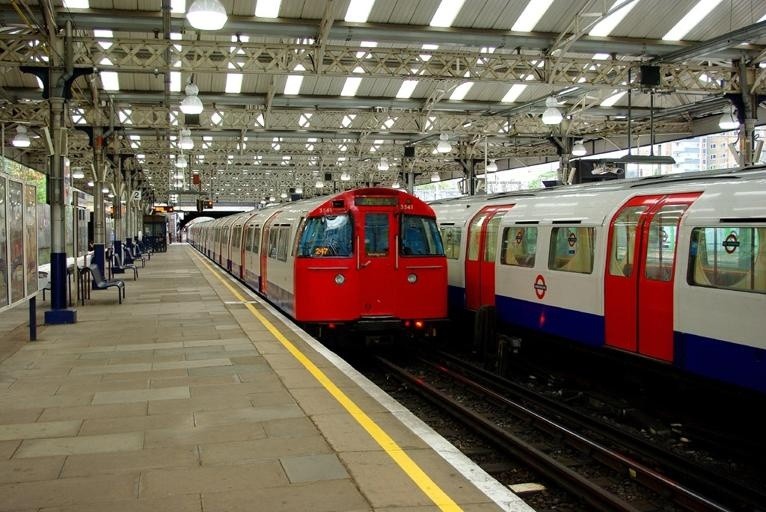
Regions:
[178,129,194,150]
[270,196,275,201]
[316,180,324,188]
[13,126,30,148]
[175,154,188,168]
[392,181,400,189]
[571,140,588,157]
[377,158,390,171]
[173,172,184,187]
[295,187,303,194]
[430,171,441,183]
[438,133,452,153]
[486,160,498,172]
[281,192,288,197]
[542,101,563,125]
[178,84,204,116]
[88,180,94,186]
[72,166,85,179]
[185,0,228,32]
[340,170,351,182]
[718,105,739,130]
[261,199,267,203]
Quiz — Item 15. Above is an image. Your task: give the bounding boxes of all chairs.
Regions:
[81,240,152,304]
